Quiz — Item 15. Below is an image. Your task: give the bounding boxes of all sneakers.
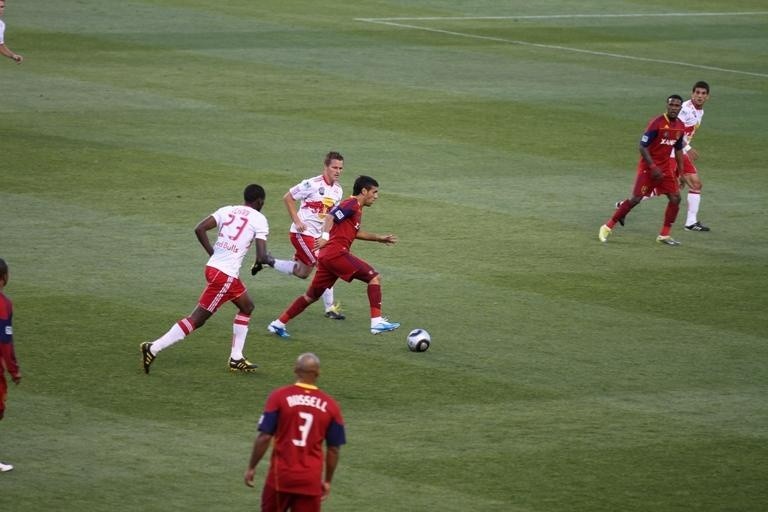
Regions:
[598,201,626,242]
[0,463,14,472]
[655,234,680,245]
[323,304,346,320]
[267,321,290,337]
[686,221,708,232]
[371,317,400,335]
[228,356,258,373]
[140,342,156,374]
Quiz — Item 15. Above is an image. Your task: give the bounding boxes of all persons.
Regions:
[598,94,687,247]
[251,152,349,320]
[1,258,23,473]
[0,0,23,65]
[245,353,345,512]
[663,81,713,232]
[268,175,401,340]
[141,182,271,376]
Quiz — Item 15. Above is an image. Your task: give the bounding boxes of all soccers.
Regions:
[407,329,430,352]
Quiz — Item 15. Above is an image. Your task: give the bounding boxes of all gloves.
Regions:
[251,262,263,276]
